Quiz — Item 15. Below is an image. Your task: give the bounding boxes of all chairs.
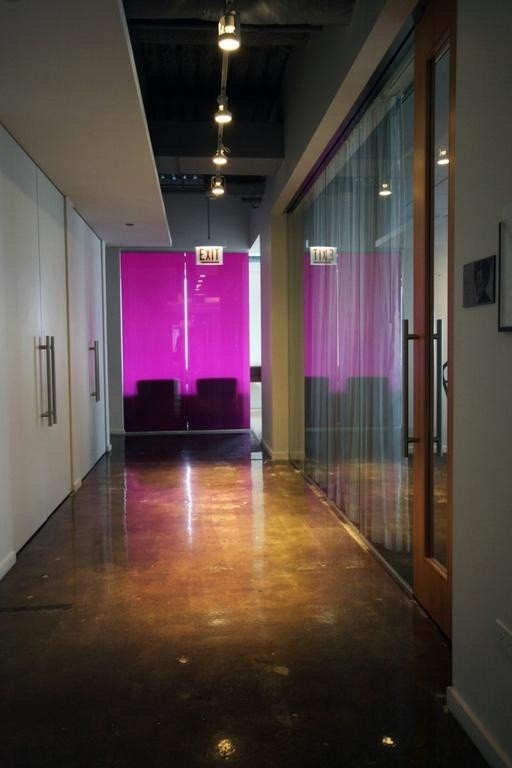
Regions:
[303,375,398,460]
[123,377,246,432]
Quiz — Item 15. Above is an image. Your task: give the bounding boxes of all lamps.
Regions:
[436,143,451,166]
[378,175,393,199]
[208,1,242,199]
